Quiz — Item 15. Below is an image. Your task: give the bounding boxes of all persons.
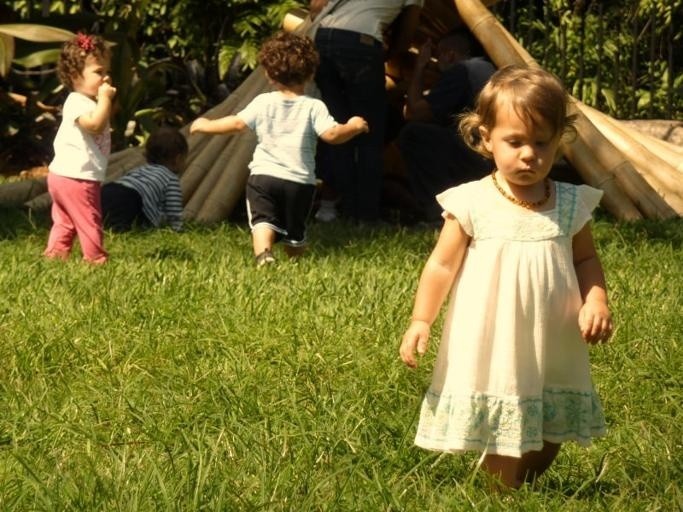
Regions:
[403,26,496,227]
[100,127,189,233]
[308,1,424,225]
[42,30,117,264]
[399,64,613,490]
[189,32,369,270]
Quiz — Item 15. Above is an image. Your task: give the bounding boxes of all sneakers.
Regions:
[312,198,338,223]
[253,247,276,272]
